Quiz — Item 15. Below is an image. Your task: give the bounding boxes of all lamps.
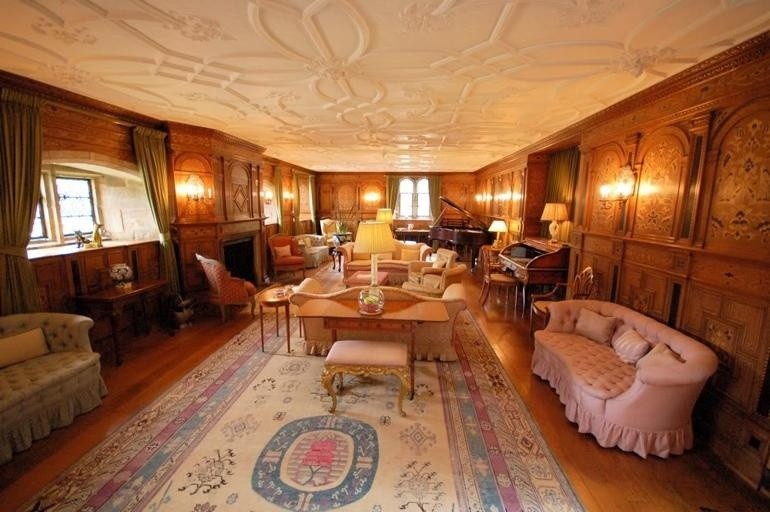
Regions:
[353,219,396,285]
[376,208,393,225]
[540,202,569,243]
[488,220,507,250]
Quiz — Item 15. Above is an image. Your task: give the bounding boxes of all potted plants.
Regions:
[332,201,359,248]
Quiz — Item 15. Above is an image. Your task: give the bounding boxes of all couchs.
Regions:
[402,264,467,298]
[408,248,459,284]
[531,299,719,459]
[336,241,433,284]
[0,312,109,468]
[289,278,468,363]
[295,234,329,268]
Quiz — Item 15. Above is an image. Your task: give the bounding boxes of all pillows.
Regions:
[0,328,51,369]
[304,238,313,247]
[372,251,393,260]
[610,323,650,363]
[274,244,292,258]
[352,248,371,260]
[431,254,448,268]
[434,279,441,290]
[574,308,618,347]
[636,343,683,375]
[400,249,420,261]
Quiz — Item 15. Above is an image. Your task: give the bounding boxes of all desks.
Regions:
[396,229,430,243]
[71,277,176,367]
[294,299,450,401]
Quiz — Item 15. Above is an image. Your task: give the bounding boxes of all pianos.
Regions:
[428,195,494,273]
[498,237,570,320]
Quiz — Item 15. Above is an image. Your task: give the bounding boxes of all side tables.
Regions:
[254,280,302,353]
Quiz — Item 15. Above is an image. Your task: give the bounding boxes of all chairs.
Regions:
[268,233,307,280]
[528,266,594,342]
[478,247,518,308]
[320,219,340,247]
[195,253,257,323]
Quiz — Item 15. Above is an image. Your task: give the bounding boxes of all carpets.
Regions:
[14,308,587,512]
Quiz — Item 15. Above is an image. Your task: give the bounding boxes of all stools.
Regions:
[320,340,412,417]
[331,248,343,272]
[479,245,504,283]
